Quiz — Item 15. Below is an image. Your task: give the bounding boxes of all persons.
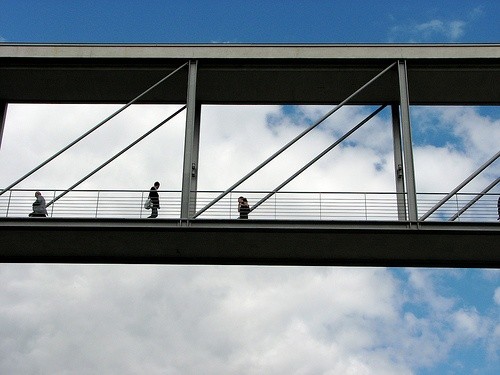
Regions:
[237,196,252,220]
[497,196,500,221]
[148,182,160,218]
[31,192,49,218]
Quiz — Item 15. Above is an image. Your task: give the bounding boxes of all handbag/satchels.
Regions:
[144,197,153,210]
